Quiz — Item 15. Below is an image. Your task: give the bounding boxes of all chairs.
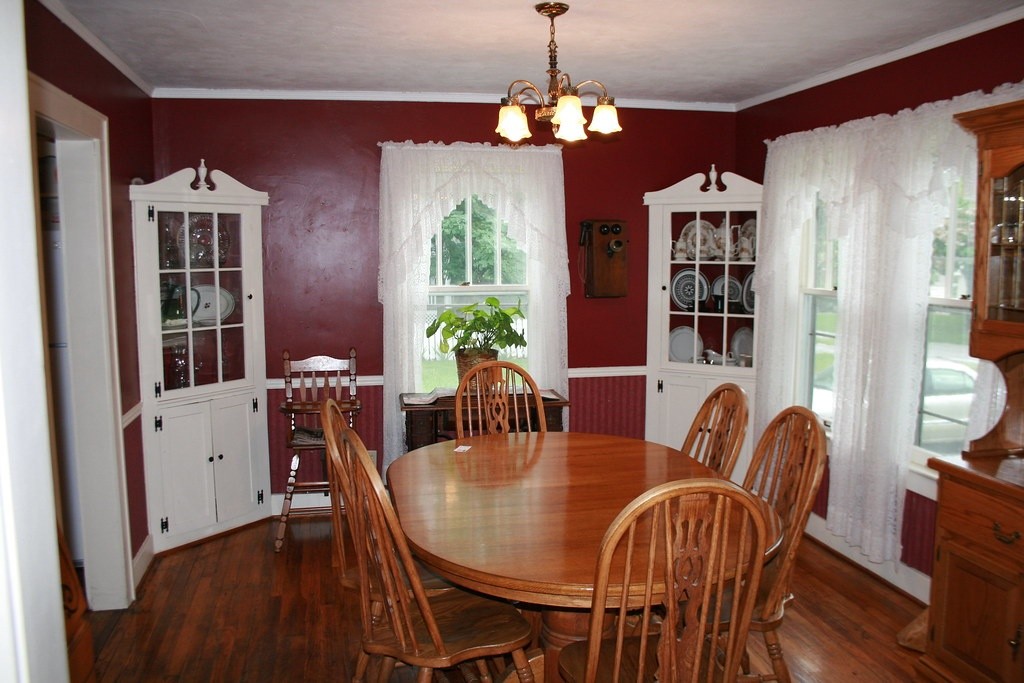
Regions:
[321,362,826,683]
[273,347,361,552]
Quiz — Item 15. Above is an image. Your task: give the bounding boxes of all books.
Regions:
[291,426,324,444]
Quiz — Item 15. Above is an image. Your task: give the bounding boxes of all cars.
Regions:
[813,356,978,446]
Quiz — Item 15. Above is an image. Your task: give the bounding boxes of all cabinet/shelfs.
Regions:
[128,159,273,554]
[919,94,1024,683]
[642,164,767,491]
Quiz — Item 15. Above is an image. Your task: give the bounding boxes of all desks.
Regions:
[400,392,573,452]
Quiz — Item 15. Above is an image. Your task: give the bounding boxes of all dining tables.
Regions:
[385,431,784,682]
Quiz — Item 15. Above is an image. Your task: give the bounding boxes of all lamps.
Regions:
[494,1,623,141]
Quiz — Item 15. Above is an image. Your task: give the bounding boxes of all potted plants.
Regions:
[426,297,525,398]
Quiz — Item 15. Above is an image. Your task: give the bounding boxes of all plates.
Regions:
[668,220,756,368]
[177,213,237,326]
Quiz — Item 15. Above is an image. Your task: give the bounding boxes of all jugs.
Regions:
[164,286,201,326]
[168,345,204,391]
